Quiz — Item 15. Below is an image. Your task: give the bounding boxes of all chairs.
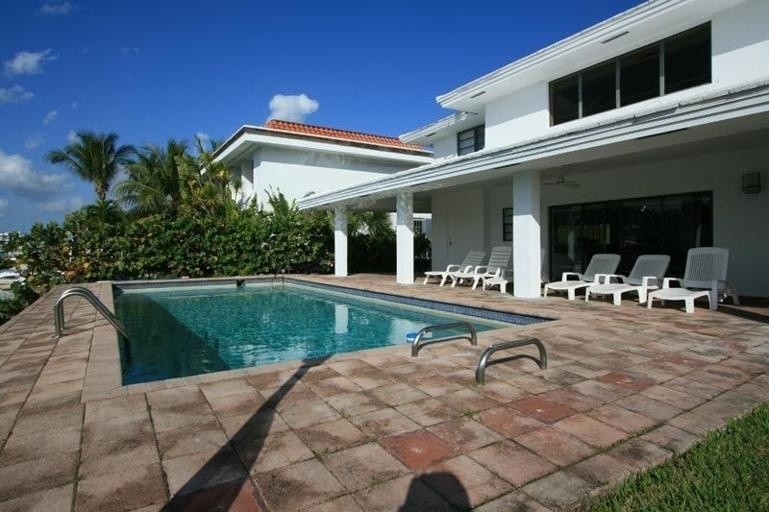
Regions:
[543,245,739,316]
[425,246,515,295]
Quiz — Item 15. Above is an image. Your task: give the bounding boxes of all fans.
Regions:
[540,174,583,189]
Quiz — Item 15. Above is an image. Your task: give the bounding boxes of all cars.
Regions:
[0,273,27,290]
